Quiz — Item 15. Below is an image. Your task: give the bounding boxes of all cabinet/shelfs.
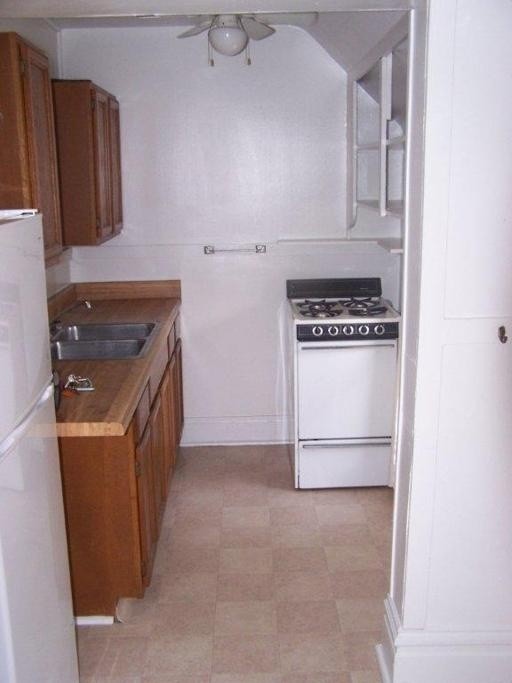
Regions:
[0,30,63,256]
[346,9,410,231]
[58,311,184,598]
[51,78,124,247]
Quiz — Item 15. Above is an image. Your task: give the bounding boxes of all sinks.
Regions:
[50,340,147,360]
[54,321,155,340]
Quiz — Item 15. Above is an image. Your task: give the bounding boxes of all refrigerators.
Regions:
[0,208,82,683]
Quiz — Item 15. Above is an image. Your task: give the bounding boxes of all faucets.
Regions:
[51,295,93,344]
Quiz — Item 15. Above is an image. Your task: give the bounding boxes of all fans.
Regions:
[176,10,318,40]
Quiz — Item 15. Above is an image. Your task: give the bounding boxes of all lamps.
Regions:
[206,26,252,68]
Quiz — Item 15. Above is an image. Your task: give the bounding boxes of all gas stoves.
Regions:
[290,297,396,319]
